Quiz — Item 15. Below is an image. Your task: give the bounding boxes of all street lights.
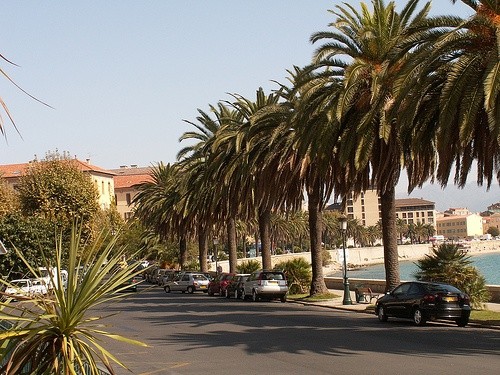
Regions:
[212,235,219,276]
[337,212,353,305]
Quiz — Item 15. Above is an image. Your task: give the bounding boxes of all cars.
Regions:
[375,282,471,326]
[0,258,288,302]
